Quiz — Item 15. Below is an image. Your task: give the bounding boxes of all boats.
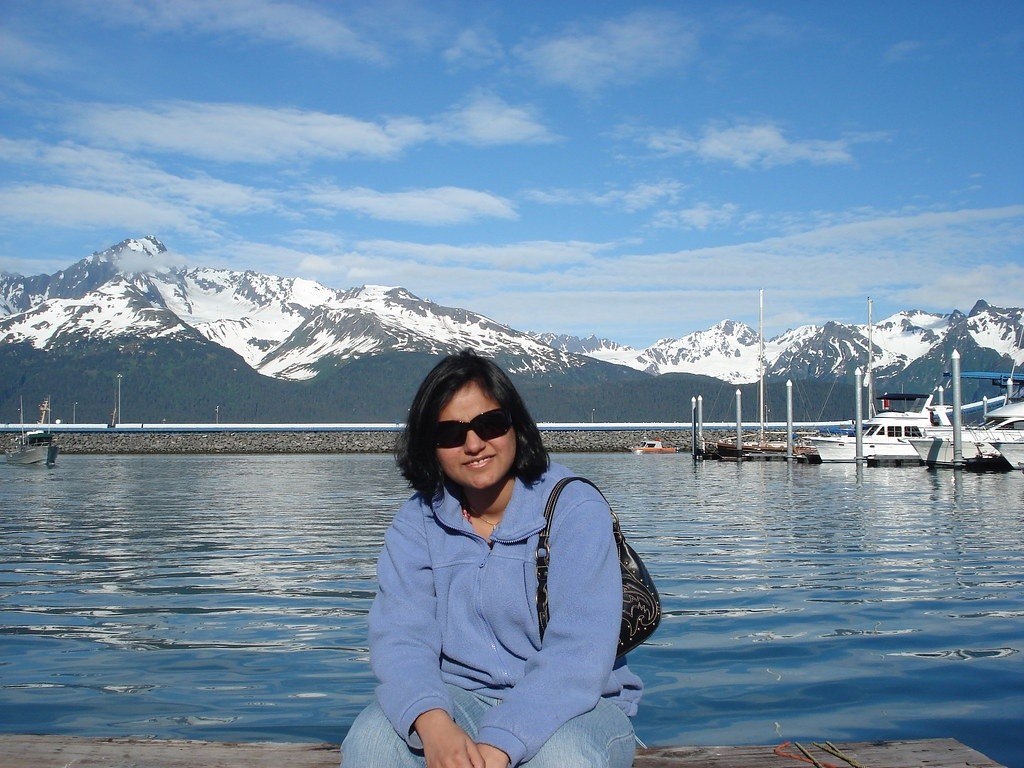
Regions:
[629,439,680,454]
[802,388,1024,474]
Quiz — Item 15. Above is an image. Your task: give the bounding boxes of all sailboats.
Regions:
[5,395,54,466]
[713,289,808,463]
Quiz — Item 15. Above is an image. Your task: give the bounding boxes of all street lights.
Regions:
[216,406,219,424]
[70,399,78,424]
[592,408,596,423]
[116,373,122,424]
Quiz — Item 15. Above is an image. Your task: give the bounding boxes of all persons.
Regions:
[340,347,644,768]
[55,417,61,424]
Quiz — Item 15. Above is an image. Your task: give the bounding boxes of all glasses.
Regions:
[429,405,514,449]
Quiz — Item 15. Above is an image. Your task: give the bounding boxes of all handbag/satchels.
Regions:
[536,476,661,667]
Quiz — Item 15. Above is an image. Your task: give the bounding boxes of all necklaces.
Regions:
[470,506,499,530]
